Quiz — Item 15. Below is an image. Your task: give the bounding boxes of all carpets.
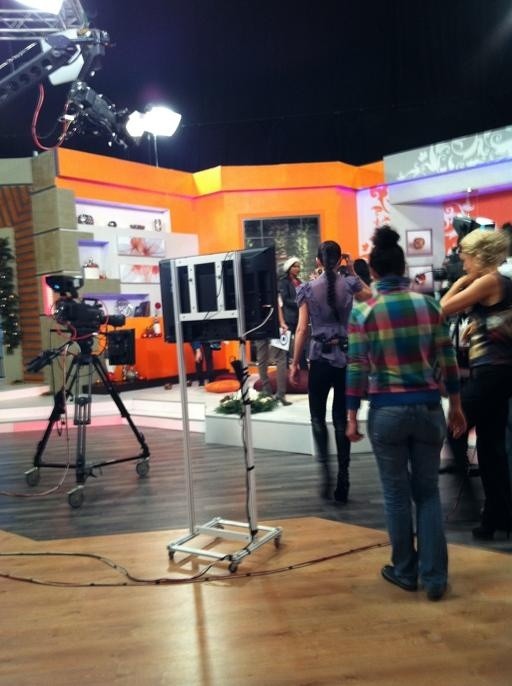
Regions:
[0,424,512,557]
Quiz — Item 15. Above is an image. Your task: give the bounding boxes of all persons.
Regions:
[289,241,371,504]
[255,257,304,407]
[346,225,468,602]
[439,221,512,544]
[190,341,216,387]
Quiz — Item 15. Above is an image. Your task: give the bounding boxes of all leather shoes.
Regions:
[381,563,419,591]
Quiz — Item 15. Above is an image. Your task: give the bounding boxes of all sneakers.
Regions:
[473,525,512,543]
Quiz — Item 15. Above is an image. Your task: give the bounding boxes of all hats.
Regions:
[284,257,300,273]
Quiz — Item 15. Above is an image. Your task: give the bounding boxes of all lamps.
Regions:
[116,104,182,149]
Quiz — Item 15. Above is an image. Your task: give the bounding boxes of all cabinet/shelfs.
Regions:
[69,199,198,317]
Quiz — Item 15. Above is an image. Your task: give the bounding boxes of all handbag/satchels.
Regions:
[445,314,472,394]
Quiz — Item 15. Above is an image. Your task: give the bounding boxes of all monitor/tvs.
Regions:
[157,246,279,343]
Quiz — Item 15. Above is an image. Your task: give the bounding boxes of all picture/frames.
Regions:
[407,229,434,258]
[408,265,434,294]
